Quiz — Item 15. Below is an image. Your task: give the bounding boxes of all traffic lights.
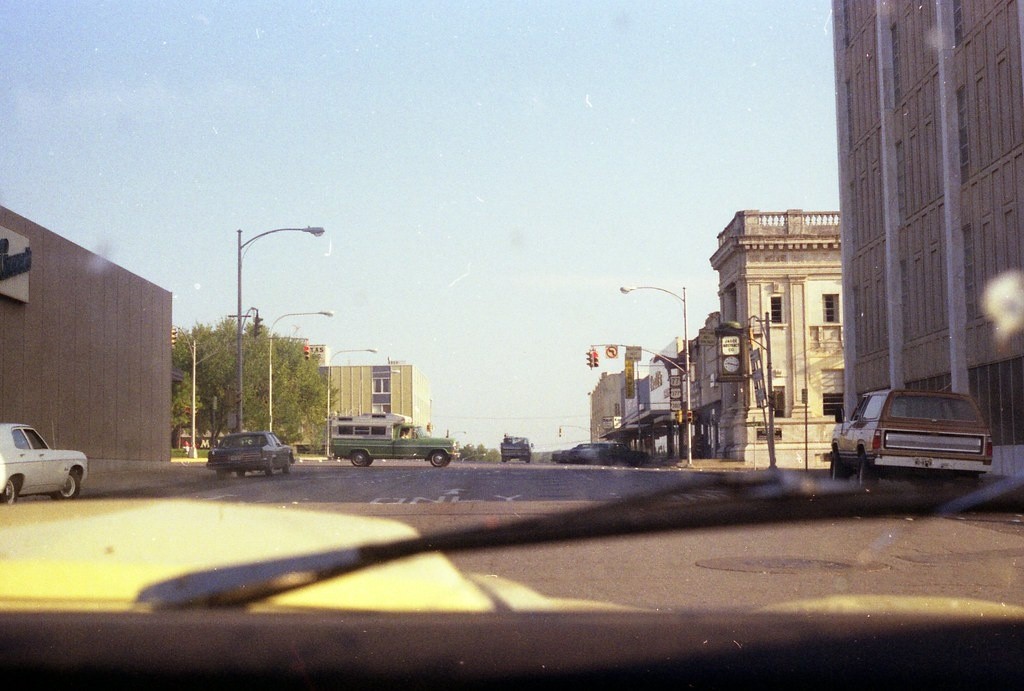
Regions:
[676,410,684,425]
[252,316,265,337]
[559,428,561,436]
[172,329,177,346]
[304,346,310,360]
[687,410,692,423]
[445,431,466,437]
[592,352,599,368]
[586,351,592,370]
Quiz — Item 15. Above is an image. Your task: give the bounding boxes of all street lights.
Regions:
[326,348,378,458]
[620,285,692,465]
[267,311,334,430]
[237,227,324,434]
[715,328,776,467]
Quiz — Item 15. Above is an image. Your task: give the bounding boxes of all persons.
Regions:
[401,428,411,439]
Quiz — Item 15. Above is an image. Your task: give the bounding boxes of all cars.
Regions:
[0,421,90,503]
[553,443,648,467]
[830,389,993,487]
[207,431,297,478]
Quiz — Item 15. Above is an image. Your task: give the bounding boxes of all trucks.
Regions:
[331,413,462,467]
[501,436,534,464]
